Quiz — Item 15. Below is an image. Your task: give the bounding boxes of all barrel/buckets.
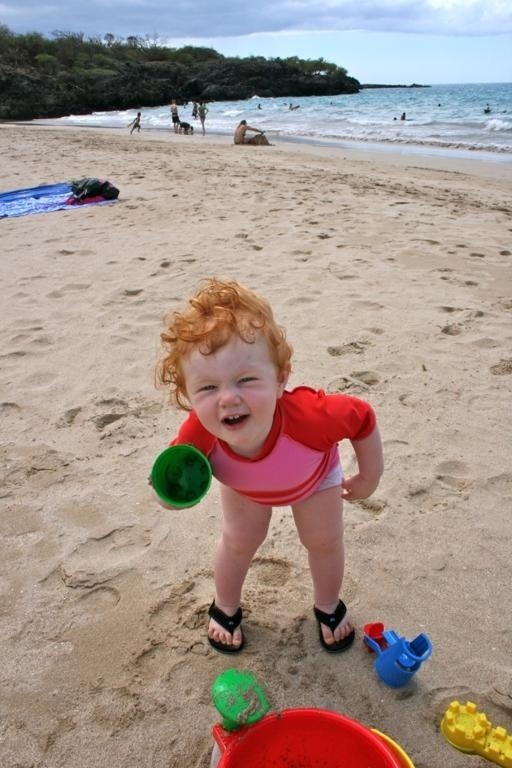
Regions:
[209,707,416,768]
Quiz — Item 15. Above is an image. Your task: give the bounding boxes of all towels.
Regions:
[0,181,120,218]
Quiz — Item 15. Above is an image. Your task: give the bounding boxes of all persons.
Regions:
[234,120,261,143]
[178,121,193,134]
[197,103,208,135]
[129,112,141,134]
[401,112,406,120]
[485,103,489,112]
[192,101,197,118]
[148,276,385,652]
[171,99,180,133]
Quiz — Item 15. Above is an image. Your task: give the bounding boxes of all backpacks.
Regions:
[70,177,120,200]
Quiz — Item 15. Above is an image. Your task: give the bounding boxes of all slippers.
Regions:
[311,599,355,653]
[206,596,245,653]
